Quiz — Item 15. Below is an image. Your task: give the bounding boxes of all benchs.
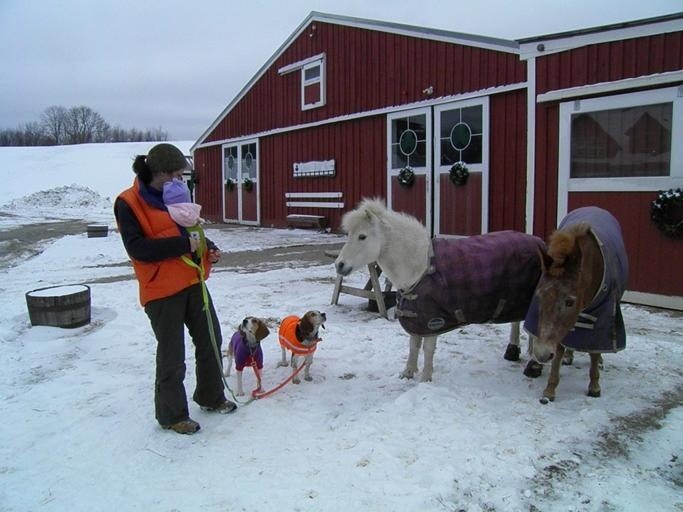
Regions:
[323,249,398,320]
[286,214,326,231]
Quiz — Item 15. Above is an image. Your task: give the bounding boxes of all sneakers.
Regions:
[200,399,237,415]
[162,417,200,434]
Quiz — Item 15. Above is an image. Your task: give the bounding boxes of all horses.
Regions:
[529,204,628,405]
[334,195,549,384]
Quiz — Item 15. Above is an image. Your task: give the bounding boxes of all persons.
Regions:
[112,142,237,439]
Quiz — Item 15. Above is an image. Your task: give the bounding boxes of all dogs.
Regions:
[279,310,327,383]
[223,316,270,397]
